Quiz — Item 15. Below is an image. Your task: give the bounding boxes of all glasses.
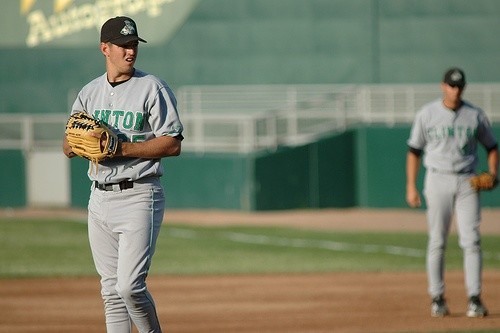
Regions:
[449,83,462,88]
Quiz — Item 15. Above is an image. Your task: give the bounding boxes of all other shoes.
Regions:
[466,297,488,317]
[432,299,447,317]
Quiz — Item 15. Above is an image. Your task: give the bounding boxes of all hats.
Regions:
[100,17,147,45]
[445,69,467,85]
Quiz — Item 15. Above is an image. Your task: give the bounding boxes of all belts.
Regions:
[95,181,133,191]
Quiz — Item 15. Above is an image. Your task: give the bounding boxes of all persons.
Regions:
[406,68,500,318]
[63,16,185,333]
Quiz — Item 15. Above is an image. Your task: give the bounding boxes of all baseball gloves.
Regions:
[65,112,120,162]
